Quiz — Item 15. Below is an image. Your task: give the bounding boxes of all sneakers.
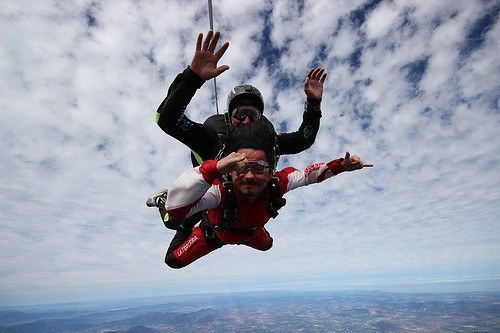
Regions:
[146,188,169,208]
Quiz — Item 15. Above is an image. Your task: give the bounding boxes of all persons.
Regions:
[147,30,328,230]
[163,142,373,269]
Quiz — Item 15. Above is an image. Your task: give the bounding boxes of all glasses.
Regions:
[240,165,270,175]
[232,108,262,123]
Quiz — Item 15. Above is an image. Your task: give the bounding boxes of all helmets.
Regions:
[227,84,265,117]
